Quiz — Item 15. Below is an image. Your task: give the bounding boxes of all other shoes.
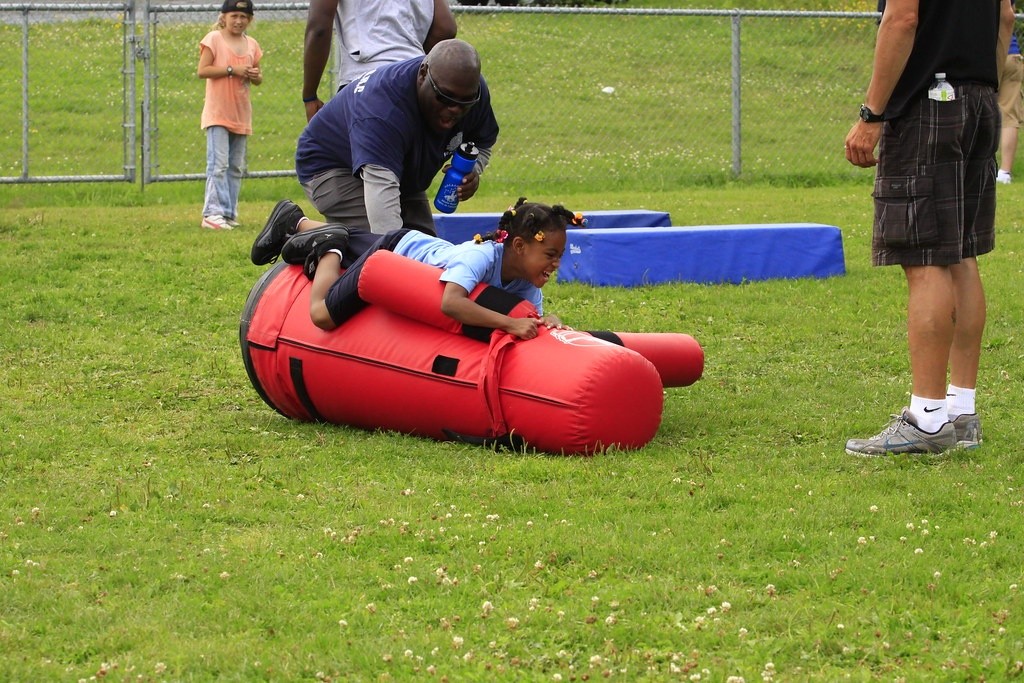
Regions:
[997,168,1011,183]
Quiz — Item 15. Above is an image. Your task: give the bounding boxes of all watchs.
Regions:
[227,66,233,76]
[859,105,886,123]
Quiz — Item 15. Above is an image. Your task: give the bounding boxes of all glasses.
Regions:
[426,64,481,109]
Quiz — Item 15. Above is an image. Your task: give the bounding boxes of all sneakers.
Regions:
[951,412,984,449]
[252,198,304,265]
[201,214,229,228]
[282,223,349,280]
[226,217,239,226]
[846,406,956,457]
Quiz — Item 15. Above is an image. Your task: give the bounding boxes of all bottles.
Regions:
[928,72,956,101]
[433,142,479,214]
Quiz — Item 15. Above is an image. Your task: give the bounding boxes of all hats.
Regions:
[222,0,253,14]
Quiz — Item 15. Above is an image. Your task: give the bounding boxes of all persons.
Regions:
[295,38,499,237]
[250,196,588,341]
[844,0,1015,457]
[996,34,1024,184]
[303,0,457,124]
[197,0,264,230]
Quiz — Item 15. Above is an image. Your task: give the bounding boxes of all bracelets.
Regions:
[303,95,316,102]
